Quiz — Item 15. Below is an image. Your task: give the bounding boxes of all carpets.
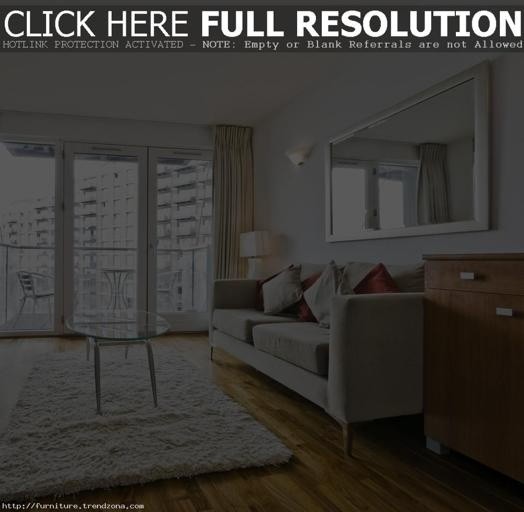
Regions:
[1,350,291,504]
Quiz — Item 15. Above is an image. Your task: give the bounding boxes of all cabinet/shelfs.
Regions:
[421,252,523,487]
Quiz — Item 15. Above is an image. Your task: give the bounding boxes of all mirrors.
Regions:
[324,58,493,241]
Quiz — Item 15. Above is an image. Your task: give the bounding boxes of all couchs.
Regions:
[205,261,426,459]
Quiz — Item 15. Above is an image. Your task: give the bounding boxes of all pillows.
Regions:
[250,262,399,330]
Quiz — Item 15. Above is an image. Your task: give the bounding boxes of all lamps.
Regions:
[240,231,272,276]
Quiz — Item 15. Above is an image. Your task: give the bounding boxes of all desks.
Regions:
[87,264,136,310]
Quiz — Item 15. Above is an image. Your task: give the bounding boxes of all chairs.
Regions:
[12,269,56,331]
[153,270,181,312]
